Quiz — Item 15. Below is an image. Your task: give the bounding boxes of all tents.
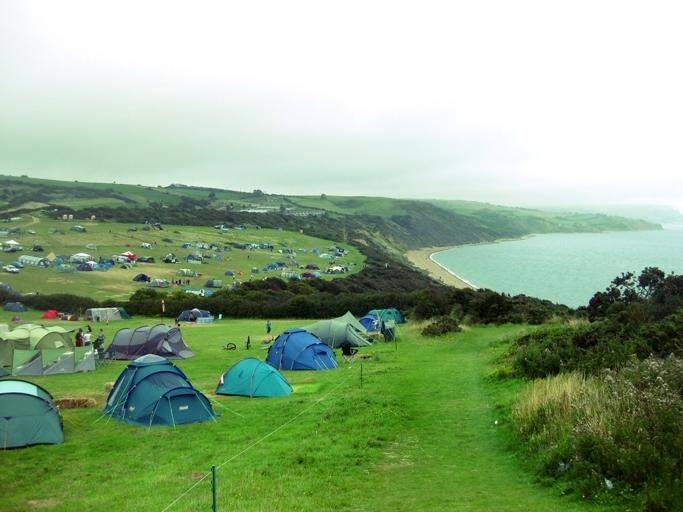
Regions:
[0,380,66,448]
[304,307,408,349]
[264,329,340,370]
[214,357,294,398]
[104,354,223,427]
[0,299,215,378]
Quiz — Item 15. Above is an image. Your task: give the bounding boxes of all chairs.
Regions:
[341,341,358,364]
